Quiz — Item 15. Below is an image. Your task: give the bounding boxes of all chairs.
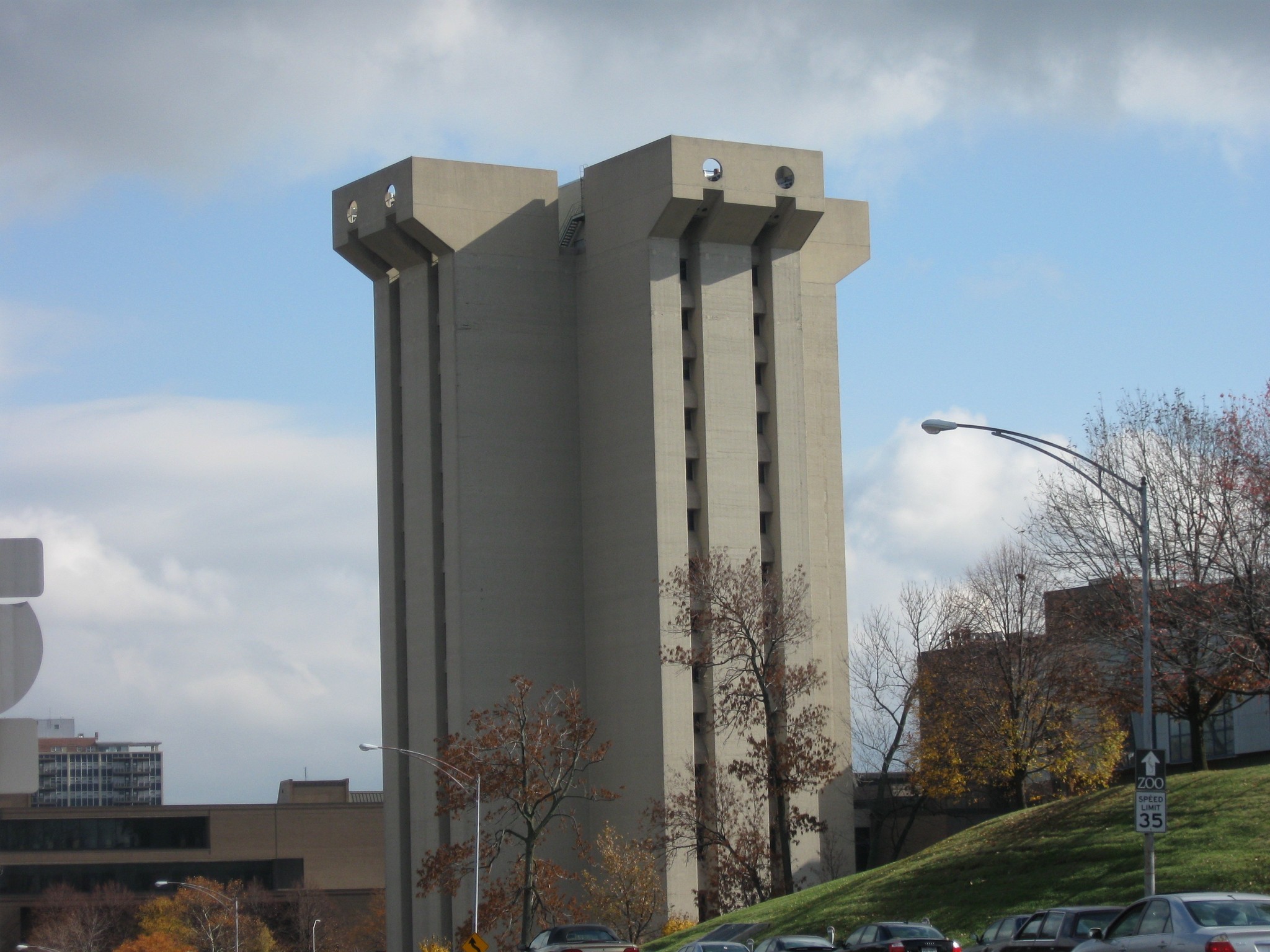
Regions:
[1215,908,1237,927]
[568,930,580,938]
[583,931,599,940]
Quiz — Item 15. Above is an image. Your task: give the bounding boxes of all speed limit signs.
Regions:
[1135,791,1167,833]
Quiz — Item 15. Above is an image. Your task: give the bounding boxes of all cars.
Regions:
[676,941,752,952]
[753,934,837,952]
[528,924,641,952]
[964,906,1127,952]
[1071,892,1270,952]
[838,922,963,952]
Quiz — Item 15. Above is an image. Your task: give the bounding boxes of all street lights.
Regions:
[358,743,481,934]
[155,879,239,952]
[922,418,1159,899]
[312,919,321,952]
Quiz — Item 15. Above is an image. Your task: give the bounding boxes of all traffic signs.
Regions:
[1136,748,1166,790]
